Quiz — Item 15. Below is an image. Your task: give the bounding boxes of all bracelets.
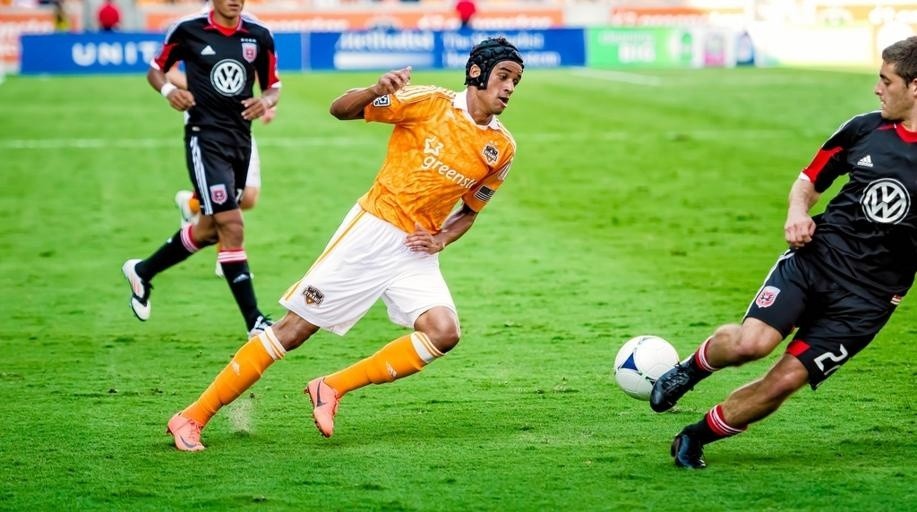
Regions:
[160,80,177,97]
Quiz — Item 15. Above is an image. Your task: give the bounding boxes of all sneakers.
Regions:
[671,425,705,468]
[215,261,253,278]
[247,313,274,341]
[175,189,200,230]
[650,353,712,412]
[304,377,341,437]
[122,259,154,321]
[166,411,206,451]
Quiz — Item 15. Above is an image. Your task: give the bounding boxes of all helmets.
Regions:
[465,38,525,90]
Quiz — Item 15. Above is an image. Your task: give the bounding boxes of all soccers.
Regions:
[614,336,680,400]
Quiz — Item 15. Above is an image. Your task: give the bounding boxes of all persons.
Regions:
[167,36,525,453]
[456,0,476,27]
[52,0,71,31]
[154,1,277,278]
[647,35,917,469]
[98,0,120,31]
[120,1,282,341]
